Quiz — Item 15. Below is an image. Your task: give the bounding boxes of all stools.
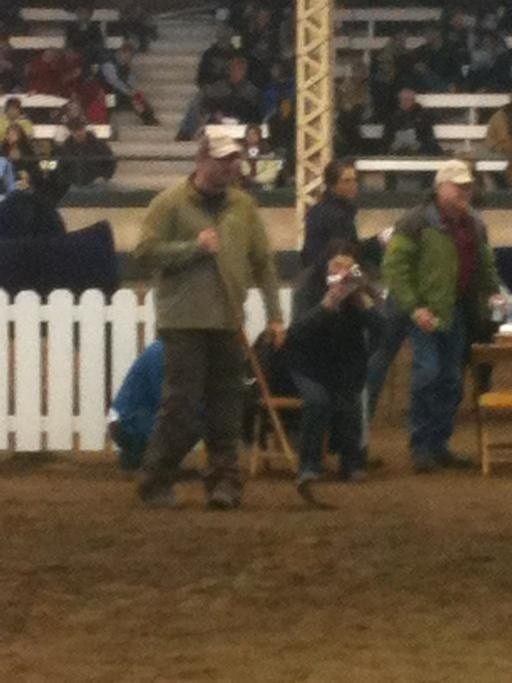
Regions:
[249,394,338,481]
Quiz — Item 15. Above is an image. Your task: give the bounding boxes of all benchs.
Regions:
[205,4,511,192]
[0,8,127,171]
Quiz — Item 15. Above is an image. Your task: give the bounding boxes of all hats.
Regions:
[435,160,476,185]
[195,124,245,159]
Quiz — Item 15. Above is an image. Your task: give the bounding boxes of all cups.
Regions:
[491,300,505,321]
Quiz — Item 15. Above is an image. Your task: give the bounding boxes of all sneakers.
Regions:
[299,460,332,484]
[136,480,180,510]
[336,464,369,482]
[207,476,243,509]
[411,445,471,472]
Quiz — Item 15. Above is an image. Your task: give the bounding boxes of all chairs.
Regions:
[471,342,512,476]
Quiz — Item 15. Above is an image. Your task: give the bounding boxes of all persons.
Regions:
[251,159,511,483]
[106,339,162,469]
[1,1,161,303]
[129,132,285,509]
[175,2,511,277]
